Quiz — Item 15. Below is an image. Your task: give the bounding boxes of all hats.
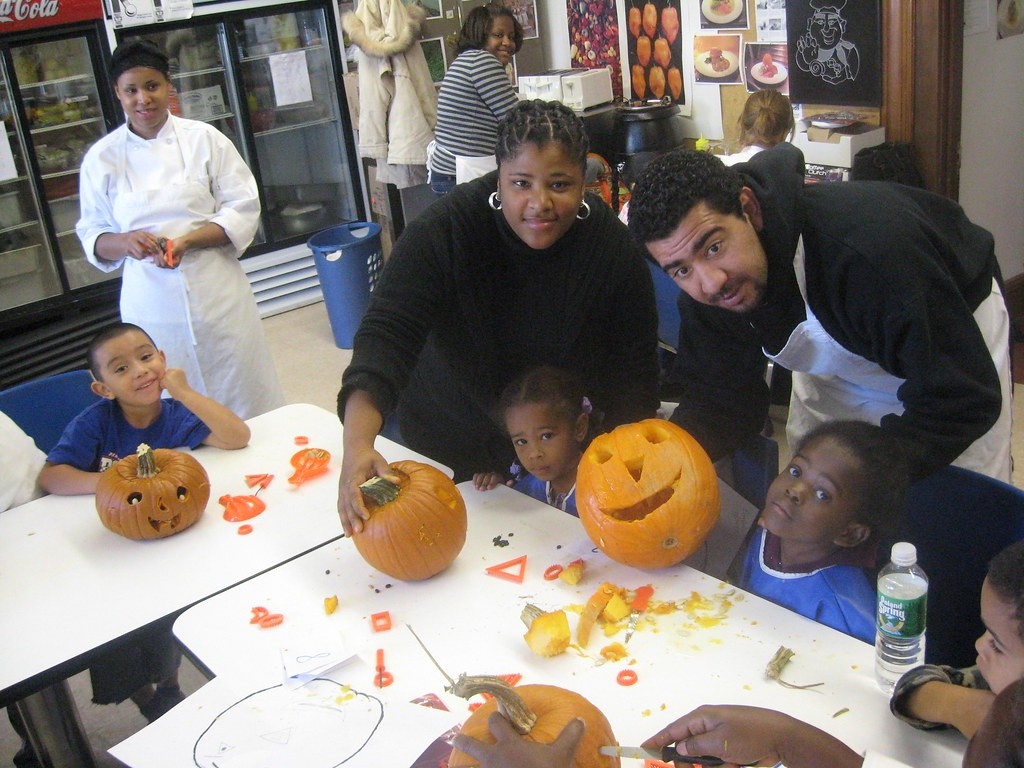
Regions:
[108,40,171,83]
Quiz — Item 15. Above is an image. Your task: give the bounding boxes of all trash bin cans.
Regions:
[305,223,385,350]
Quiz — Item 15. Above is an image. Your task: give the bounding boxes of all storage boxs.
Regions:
[789,117,886,168]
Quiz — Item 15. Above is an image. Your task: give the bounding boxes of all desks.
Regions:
[1,404,972,768]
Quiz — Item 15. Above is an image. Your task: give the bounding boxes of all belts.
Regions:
[431,173,456,181]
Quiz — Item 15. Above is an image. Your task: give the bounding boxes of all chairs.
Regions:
[880,463,1023,666]
[725,436,778,587]
[0,368,99,451]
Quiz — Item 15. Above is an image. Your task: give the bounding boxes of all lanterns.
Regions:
[574,419,722,569]
[95,443,210,540]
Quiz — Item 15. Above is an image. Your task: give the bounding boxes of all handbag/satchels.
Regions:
[851,141,926,188]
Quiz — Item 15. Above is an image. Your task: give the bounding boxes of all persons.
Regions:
[426,4,524,203]
[76,38,262,422]
[739,421,908,647]
[455,678,1024,768]
[712,90,794,167]
[37,322,250,724]
[891,539,1023,741]
[628,150,1015,528]
[335,99,659,537]
[472,365,593,519]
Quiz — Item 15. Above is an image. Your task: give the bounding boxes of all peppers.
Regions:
[566,0,621,97]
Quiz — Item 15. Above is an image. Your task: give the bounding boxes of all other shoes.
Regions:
[157,682,187,705]
[141,692,171,723]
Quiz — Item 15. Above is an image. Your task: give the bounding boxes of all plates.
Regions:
[750,61,788,84]
[701,0,743,24]
[694,50,739,78]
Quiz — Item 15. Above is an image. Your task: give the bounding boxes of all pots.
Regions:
[609,96,684,159]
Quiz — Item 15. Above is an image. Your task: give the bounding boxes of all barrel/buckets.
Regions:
[307,222,384,350]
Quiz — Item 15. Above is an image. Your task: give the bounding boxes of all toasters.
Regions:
[516,66,616,113]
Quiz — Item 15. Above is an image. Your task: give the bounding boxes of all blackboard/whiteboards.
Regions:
[785,0,883,108]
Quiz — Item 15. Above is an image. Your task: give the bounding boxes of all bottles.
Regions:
[872,542,930,693]
[695,132,710,152]
